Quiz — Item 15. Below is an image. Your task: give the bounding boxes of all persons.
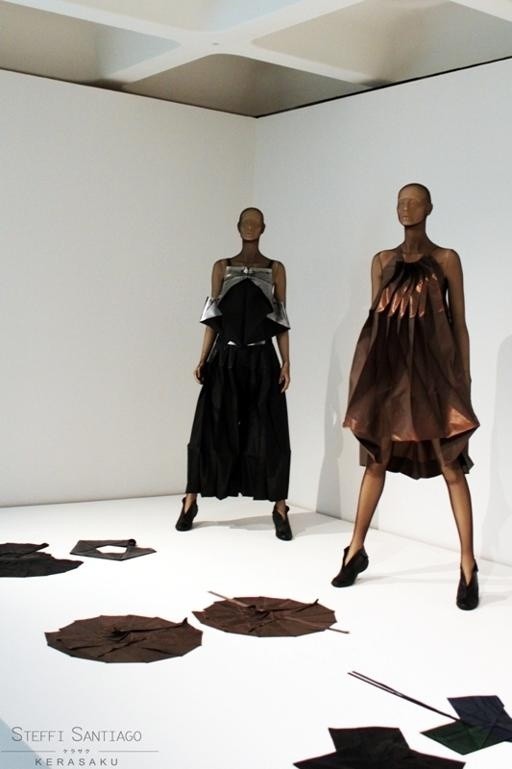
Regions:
[176,207,292,540]
[331,183,480,610]
